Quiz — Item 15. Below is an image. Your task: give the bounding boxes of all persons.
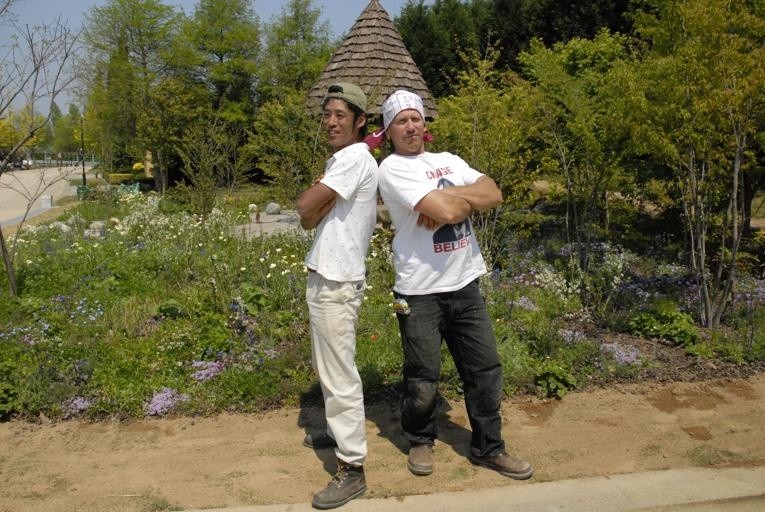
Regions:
[376,88,534,482]
[295,80,380,509]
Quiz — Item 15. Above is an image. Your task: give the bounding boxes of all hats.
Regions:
[382,89,425,132]
[321,82,368,113]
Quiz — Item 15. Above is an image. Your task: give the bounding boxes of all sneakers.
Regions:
[468,446,534,480]
[311,458,368,509]
[407,442,435,475]
[303,428,338,449]
